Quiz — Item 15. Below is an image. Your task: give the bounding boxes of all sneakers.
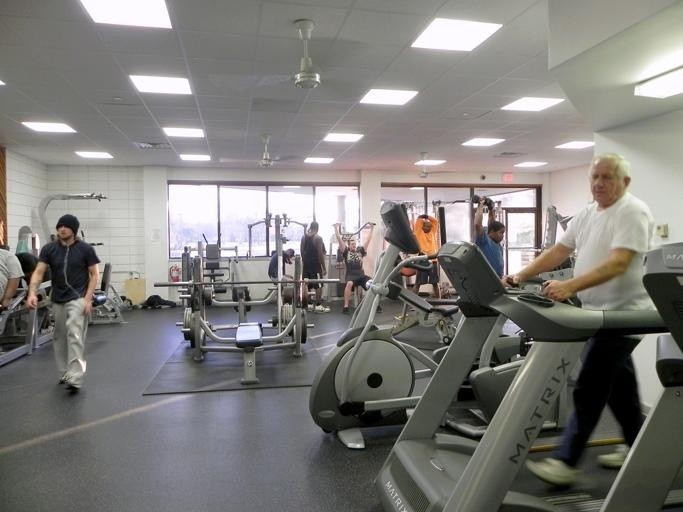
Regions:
[525,457,584,486]
[307,303,331,313]
[377,305,382,311]
[596,444,631,468]
[343,307,349,314]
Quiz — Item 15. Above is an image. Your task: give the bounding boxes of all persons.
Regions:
[0,214,101,392]
[267,248,295,287]
[334,223,382,313]
[473,197,506,280]
[413,214,442,299]
[300,222,331,313]
[501,151,655,488]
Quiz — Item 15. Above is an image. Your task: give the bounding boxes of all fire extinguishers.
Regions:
[170,264,179,282]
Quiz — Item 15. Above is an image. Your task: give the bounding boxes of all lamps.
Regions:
[634,67,683,99]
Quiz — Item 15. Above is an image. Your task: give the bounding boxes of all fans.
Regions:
[219,20,457,179]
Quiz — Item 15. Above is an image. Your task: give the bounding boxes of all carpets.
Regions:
[142,323,322,396]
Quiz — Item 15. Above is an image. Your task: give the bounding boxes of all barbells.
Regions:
[154,254,340,308]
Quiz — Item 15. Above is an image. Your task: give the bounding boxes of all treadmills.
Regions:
[598,241,682,511]
[373,241,682,511]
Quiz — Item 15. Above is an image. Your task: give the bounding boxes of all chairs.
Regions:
[204,262,226,292]
[94,263,124,304]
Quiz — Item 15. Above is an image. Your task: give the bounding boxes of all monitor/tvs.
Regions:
[380,201,420,254]
[436,240,507,305]
[642,242,683,353]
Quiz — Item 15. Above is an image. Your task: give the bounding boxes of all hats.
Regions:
[56,215,79,236]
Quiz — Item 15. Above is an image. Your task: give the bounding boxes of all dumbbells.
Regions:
[472,195,495,210]
